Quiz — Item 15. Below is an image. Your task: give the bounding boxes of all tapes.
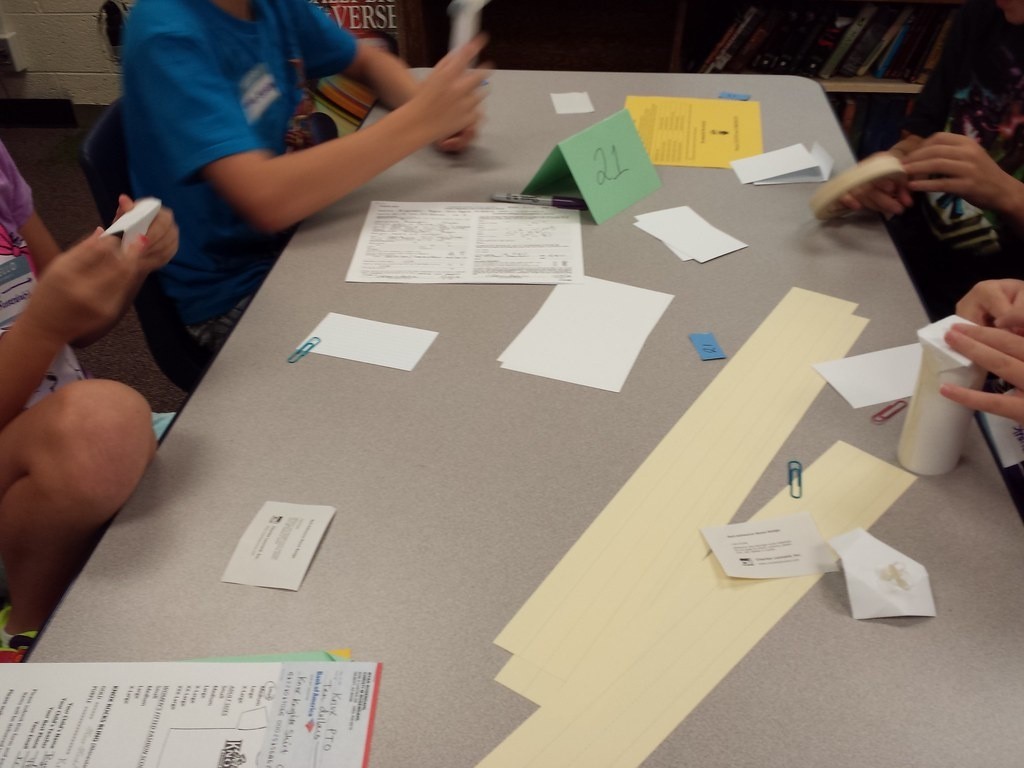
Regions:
[809,154,908,224]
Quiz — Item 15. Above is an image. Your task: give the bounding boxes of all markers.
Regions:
[490,191,587,212]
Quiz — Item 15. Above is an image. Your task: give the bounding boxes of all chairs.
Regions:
[78,91,211,394]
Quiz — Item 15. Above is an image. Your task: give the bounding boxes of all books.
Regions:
[691,0,964,159]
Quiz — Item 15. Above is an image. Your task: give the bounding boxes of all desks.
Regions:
[20,61,1024,767]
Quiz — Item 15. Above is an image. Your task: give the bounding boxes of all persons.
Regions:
[122,0,488,347]
[837,0,1024,321]
[940,278,1024,523]
[0,137,179,663]
